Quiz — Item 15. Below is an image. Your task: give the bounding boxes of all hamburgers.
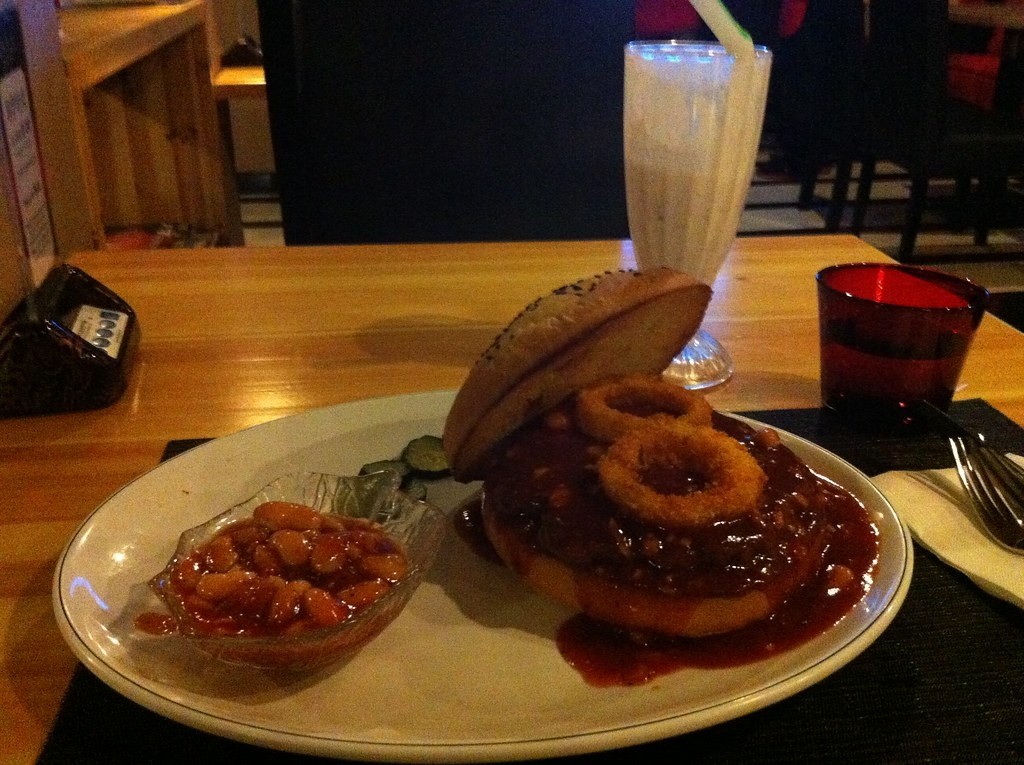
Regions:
[442,264,820,636]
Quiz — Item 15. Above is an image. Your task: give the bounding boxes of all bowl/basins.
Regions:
[140,464,447,670]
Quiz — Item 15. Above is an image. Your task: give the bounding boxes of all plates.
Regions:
[49,388,915,765]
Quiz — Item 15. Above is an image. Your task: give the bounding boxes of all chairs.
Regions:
[256,1,637,245]
[852,0,1024,261]
[797,0,869,233]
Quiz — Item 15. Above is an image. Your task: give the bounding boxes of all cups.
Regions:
[815,262,986,431]
[622,34,773,387]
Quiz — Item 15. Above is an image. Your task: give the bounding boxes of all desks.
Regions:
[0,235,1024,764]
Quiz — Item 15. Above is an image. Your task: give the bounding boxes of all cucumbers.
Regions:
[359,434,454,501]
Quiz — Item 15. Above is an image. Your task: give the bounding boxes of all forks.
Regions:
[947,433,1024,558]
